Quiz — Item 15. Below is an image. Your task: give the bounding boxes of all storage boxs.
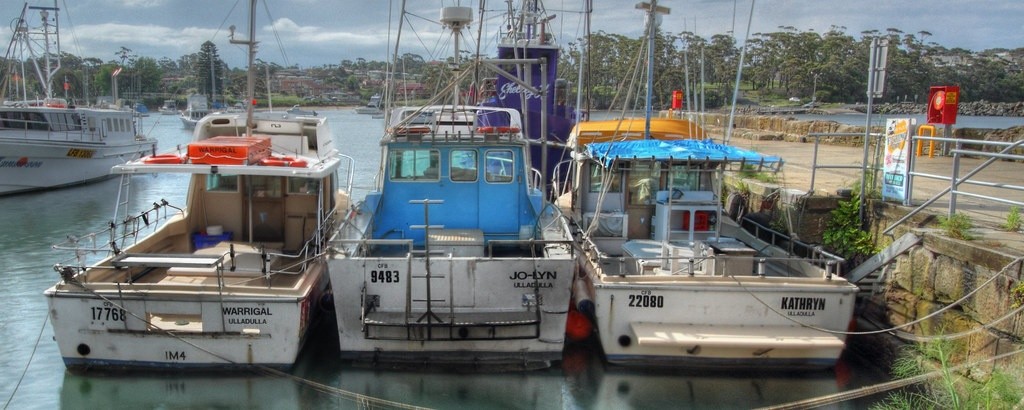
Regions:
[426,229,484,259]
[193,233,232,249]
[683,212,708,231]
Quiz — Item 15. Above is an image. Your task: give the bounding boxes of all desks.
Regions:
[656,200,722,242]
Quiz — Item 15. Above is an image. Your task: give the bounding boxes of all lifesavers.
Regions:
[143,153,181,165]
[476,127,521,134]
[387,126,431,134]
[259,156,308,167]
[46,103,65,108]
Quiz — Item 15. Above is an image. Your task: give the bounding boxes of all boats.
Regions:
[405,0,594,195]
[0,1,157,199]
[40,0,357,378]
[83,62,245,130]
[284,93,389,118]
[326,0,575,376]
[553,0,861,377]
